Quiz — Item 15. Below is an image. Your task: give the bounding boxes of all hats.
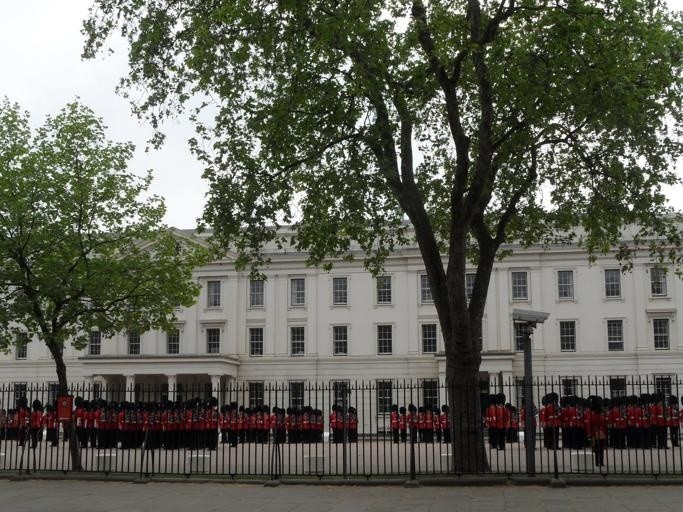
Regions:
[390,403,450,411]
[479,393,517,410]
[72,396,220,412]
[541,392,679,411]
[220,401,320,411]
[17,397,58,410]
[332,405,357,411]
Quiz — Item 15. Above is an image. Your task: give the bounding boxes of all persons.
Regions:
[219,402,325,447]
[391,403,452,444]
[2,395,220,451]
[328,404,359,443]
[483,393,682,450]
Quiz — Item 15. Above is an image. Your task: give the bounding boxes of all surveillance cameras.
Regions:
[513,308,551,323]
